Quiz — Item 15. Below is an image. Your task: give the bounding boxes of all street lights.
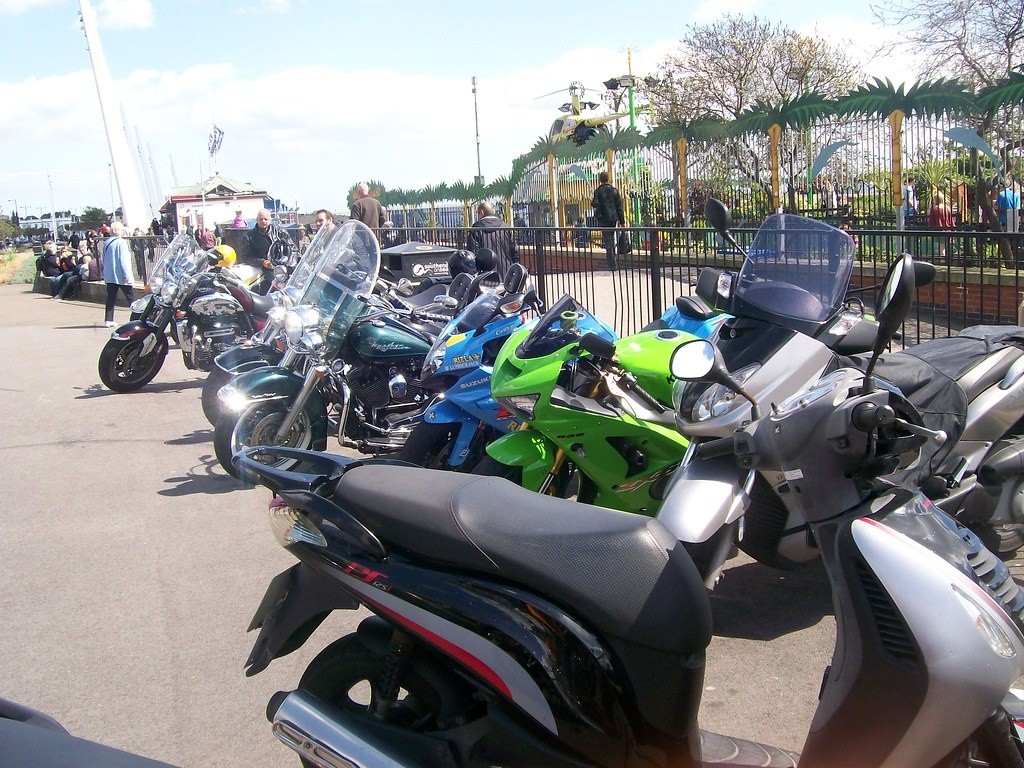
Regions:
[20,206,31,227]
[787,66,813,206]
[604,75,661,248]
[8,200,18,214]
[37,207,47,237]
[471,75,483,201]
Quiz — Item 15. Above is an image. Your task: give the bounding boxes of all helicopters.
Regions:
[534,87,660,147]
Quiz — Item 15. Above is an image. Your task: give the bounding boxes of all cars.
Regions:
[0,237,39,248]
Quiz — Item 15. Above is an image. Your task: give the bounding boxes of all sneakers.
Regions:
[105,321,118,328]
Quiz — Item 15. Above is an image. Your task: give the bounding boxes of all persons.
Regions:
[102,222,136,328]
[903,178,919,233]
[276,219,442,265]
[837,216,858,260]
[927,191,957,262]
[316,210,334,236]
[0,224,111,254]
[822,178,840,218]
[572,217,591,248]
[37,241,104,300]
[349,182,385,248]
[591,172,625,270]
[131,218,222,280]
[233,210,248,228]
[467,203,518,285]
[241,208,290,293]
[983,176,1021,268]
[514,213,527,243]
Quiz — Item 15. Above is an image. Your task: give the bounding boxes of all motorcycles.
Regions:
[97,216,735,517]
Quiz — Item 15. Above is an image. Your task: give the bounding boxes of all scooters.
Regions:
[655,197,1024,593]
[232,254,1024,768]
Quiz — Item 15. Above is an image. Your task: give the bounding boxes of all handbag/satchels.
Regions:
[618,231,631,254]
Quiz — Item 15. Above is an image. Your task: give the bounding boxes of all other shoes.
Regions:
[51,294,62,300]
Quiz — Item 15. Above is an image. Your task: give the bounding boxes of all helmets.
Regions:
[213,244,236,268]
[266,239,293,266]
[449,249,477,279]
